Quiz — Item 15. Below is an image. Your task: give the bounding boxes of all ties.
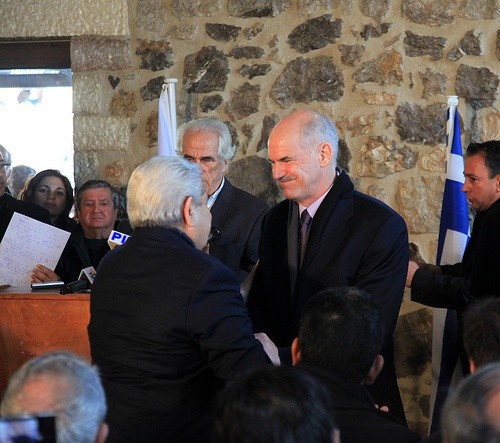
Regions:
[300,210,311,249]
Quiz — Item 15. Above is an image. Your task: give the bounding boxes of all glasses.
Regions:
[0,163,11,172]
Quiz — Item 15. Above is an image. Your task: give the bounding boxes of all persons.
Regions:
[405,140,500,443]
[462,296,500,373]
[0,108,409,443]
[440,361,500,443]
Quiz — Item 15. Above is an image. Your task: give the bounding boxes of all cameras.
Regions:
[0,417,55,443]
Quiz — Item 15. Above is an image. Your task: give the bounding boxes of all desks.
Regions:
[0,287,92,392]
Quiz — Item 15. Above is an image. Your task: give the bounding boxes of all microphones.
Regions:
[205,226,221,242]
[108,221,134,250]
[59,266,99,294]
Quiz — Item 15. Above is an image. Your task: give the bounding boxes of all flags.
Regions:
[155,79,178,161]
[427,95,471,443]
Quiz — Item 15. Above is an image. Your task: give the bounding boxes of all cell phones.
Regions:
[31,280,64,289]
